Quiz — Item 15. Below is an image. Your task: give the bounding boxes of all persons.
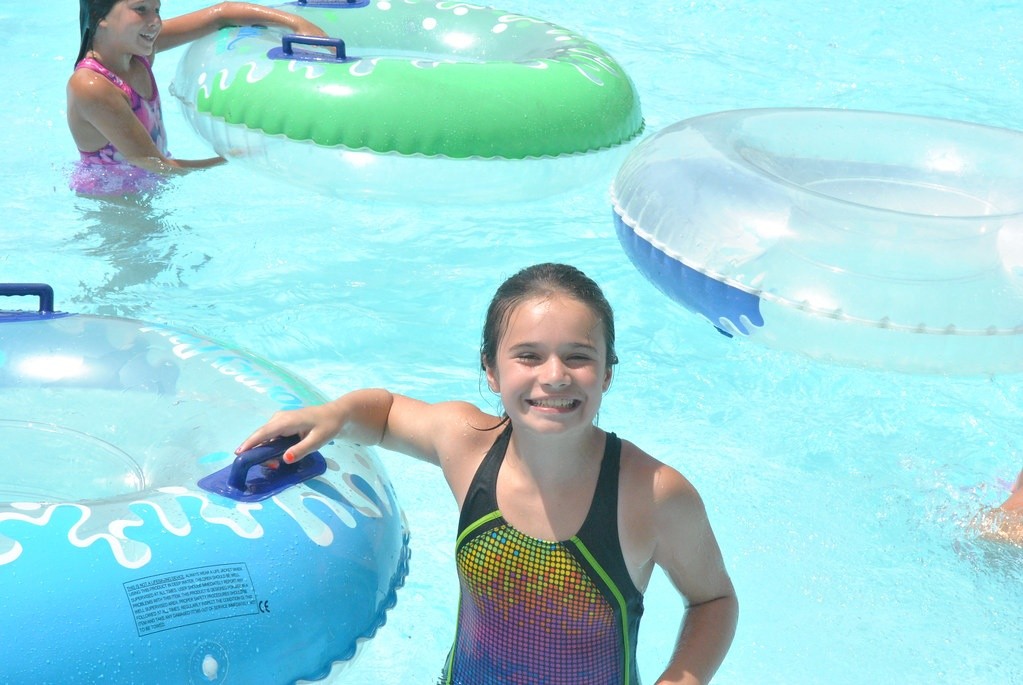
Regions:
[234,262,739,685]
[66,0,339,200]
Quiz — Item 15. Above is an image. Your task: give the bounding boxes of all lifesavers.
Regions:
[0,282,414,685]
[606,104,1023,377]
[165,0,649,210]
